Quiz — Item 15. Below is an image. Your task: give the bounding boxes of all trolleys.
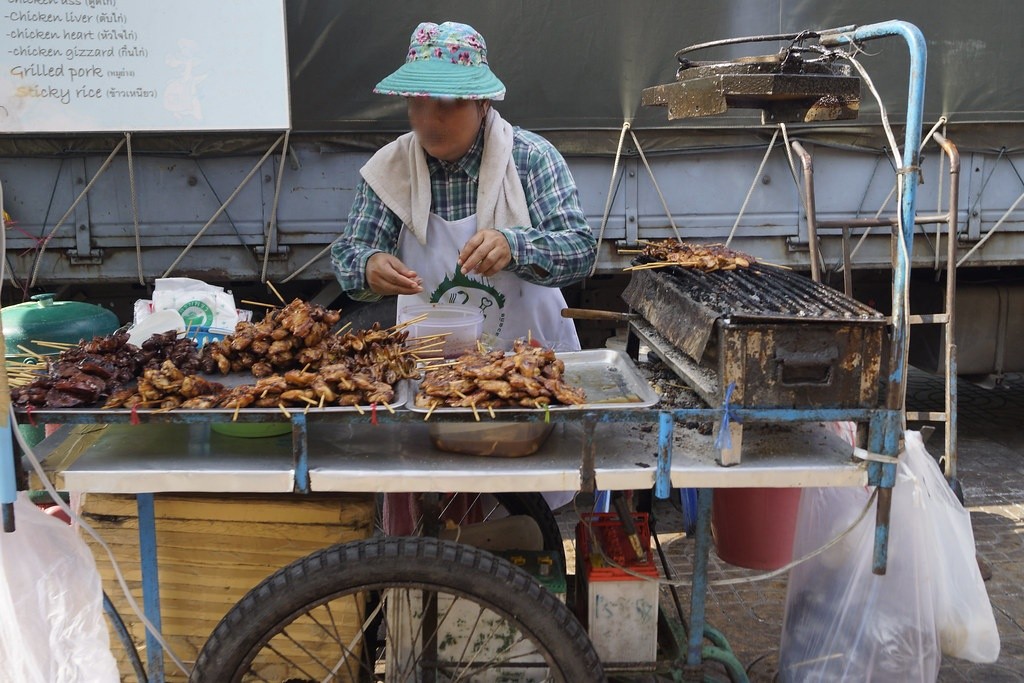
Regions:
[1,19,926,683]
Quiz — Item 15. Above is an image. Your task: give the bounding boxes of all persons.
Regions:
[328,21,598,535]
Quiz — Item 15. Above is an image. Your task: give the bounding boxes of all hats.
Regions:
[373,22,507,100]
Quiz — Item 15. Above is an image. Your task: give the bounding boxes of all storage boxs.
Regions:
[385,548,567,683]
[77,495,373,683]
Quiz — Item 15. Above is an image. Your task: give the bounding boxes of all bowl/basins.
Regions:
[399,303,485,362]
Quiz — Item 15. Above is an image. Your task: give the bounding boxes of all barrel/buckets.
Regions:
[710,488,800,572]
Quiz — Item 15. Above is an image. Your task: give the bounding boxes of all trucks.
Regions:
[1,0,1024,389]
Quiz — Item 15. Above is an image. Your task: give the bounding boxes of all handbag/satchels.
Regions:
[779,429,1000,683]
